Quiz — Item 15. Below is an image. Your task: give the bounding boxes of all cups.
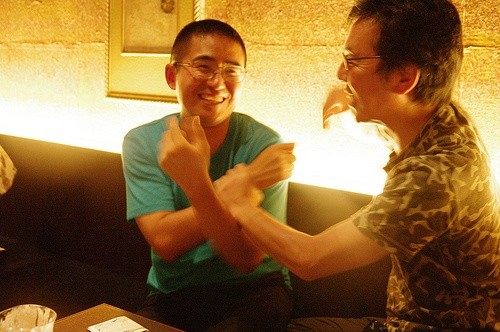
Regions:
[1,304,57,332]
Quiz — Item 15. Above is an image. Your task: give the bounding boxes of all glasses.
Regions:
[173,62,246,83]
[342,52,382,70]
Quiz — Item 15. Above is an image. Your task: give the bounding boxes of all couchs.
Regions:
[0,133,392,332]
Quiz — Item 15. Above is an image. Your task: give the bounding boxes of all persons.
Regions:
[122,19,295,331]
[214,0,500,331]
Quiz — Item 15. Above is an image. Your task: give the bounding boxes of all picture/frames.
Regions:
[106,0,205,103]
